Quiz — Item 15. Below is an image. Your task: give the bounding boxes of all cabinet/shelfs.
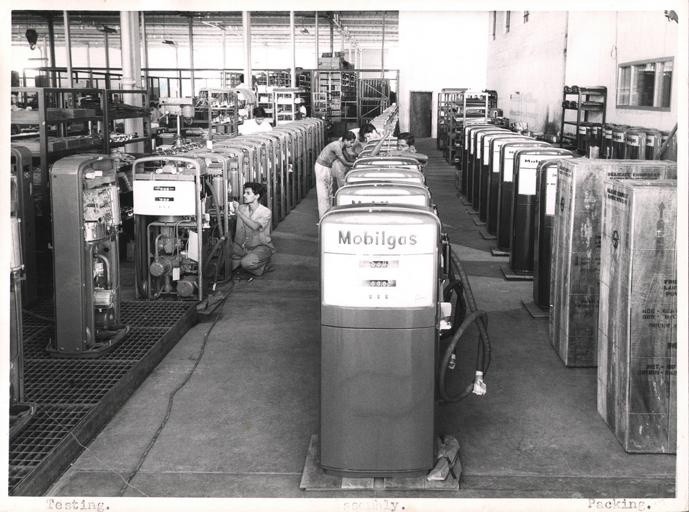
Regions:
[560,86,607,153]
[11,67,391,300]
[438,88,498,164]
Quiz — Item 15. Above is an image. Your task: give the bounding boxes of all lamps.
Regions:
[664,10,678,24]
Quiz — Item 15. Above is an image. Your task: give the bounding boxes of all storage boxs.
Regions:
[319,57,344,70]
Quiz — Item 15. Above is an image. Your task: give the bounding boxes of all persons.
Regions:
[235,73,253,115]
[359,123,379,144]
[315,131,357,226]
[332,128,362,190]
[232,182,277,276]
[298,104,307,119]
[397,132,414,153]
[239,106,271,135]
